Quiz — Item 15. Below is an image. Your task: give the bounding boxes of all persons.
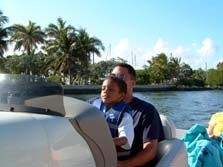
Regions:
[90,75,134,161]
[87,63,166,167]
[208,110,223,142]
